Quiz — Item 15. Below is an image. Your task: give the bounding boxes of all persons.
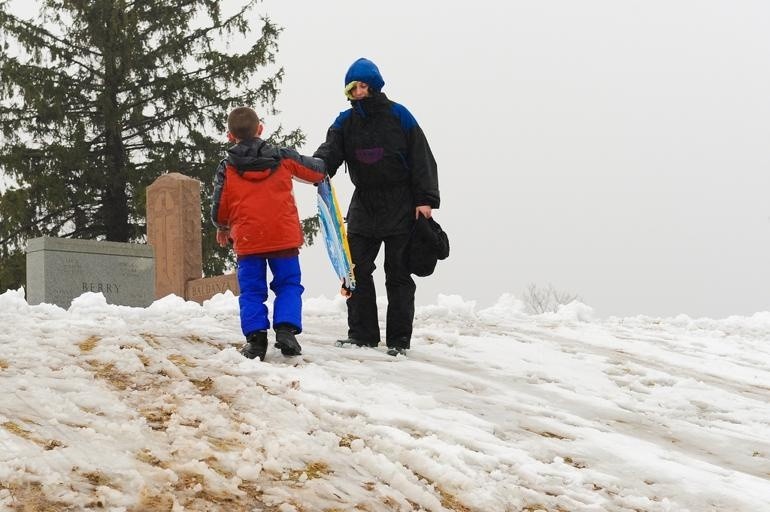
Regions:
[211,107,326,361]
[313,57,441,356]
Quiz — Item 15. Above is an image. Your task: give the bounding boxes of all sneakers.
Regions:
[337,338,378,348]
[387,346,406,356]
[275,330,301,356]
[241,342,266,361]
